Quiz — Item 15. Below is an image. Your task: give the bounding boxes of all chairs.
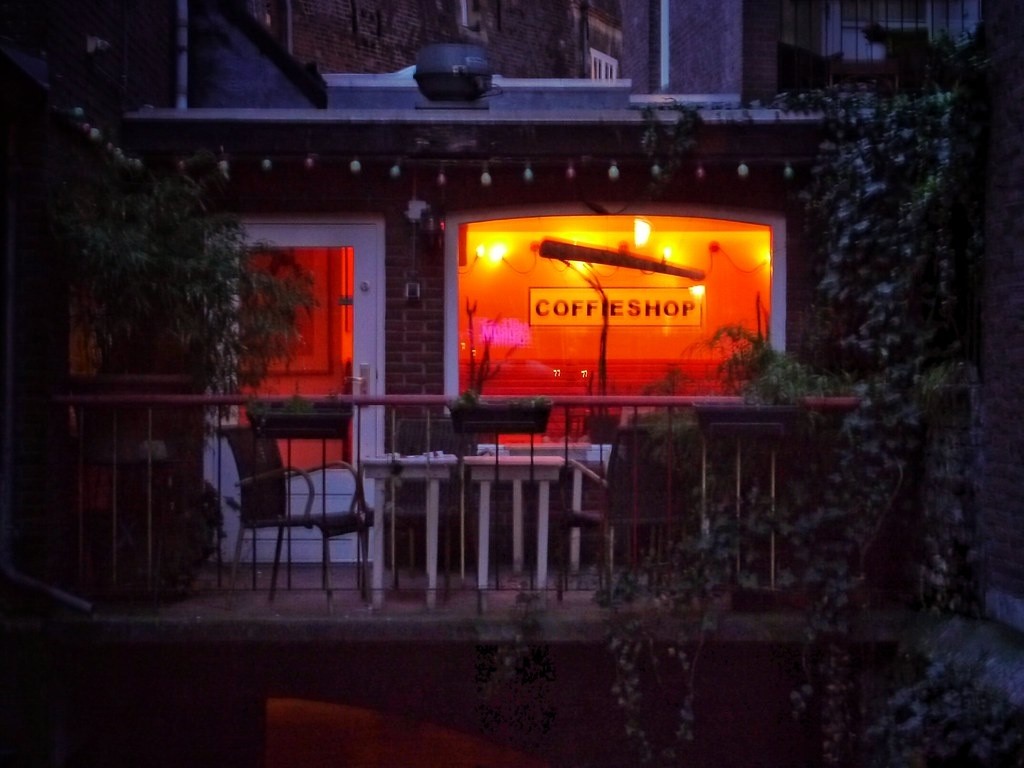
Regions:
[219,425,375,599]
[557,423,701,602]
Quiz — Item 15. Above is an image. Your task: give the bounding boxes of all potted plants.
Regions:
[449,389,550,432]
[249,392,354,439]
[698,341,818,436]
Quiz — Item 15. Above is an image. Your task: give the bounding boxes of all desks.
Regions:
[360,452,582,607]
